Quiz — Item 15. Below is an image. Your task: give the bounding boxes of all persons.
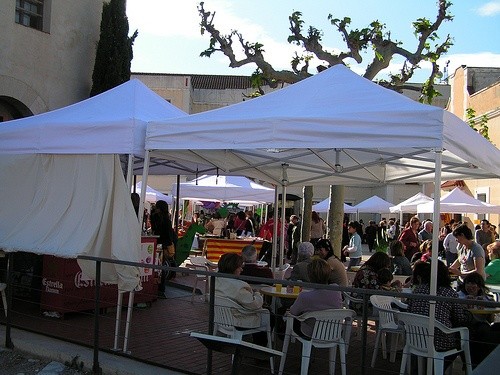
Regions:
[209,252,276,359]
[239,246,273,304]
[276,259,343,342]
[292,242,320,283]
[311,212,326,244]
[172,209,300,267]
[131,193,174,299]
[342,215,500,375]
[315,239,348,285]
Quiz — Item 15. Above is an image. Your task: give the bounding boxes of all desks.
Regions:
[467,308,500,322]
[261,287,300,369]
[207,239,263,262]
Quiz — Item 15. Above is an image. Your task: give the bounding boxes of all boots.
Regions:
[159,277,168,299]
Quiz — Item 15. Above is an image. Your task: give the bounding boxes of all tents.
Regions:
[312,196,358,220]
[417,187,500,215]
[390,193,433,226]
[0,74,279,352]
[122,65,500,374]
[172,174,275,224]
[131,180,172,204]
[349,196,395,220]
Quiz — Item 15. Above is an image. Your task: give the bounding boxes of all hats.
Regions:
[298,242,314,256]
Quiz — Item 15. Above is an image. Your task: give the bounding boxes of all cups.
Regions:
[294,286,299,294]
[275,284,282,293]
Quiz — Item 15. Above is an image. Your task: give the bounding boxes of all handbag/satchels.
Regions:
[164,241,175,257]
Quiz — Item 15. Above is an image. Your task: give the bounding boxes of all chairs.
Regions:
[207,264,472,375]
[0,250,8,317]
[191,257,218,303]
